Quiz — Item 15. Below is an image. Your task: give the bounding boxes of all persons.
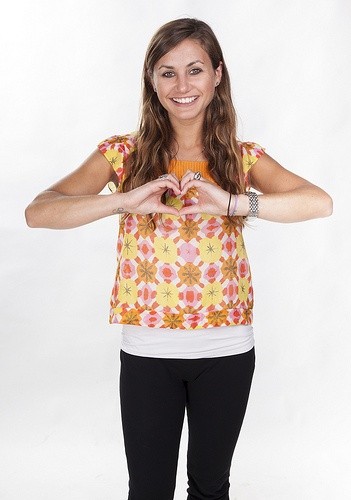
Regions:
[24,18,333,500]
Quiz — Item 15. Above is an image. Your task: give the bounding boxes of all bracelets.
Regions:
[243,192,259,218]
[227,192,238,218]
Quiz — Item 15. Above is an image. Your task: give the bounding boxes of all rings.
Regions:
[194,171,201,181]
[159,174,168,180]
